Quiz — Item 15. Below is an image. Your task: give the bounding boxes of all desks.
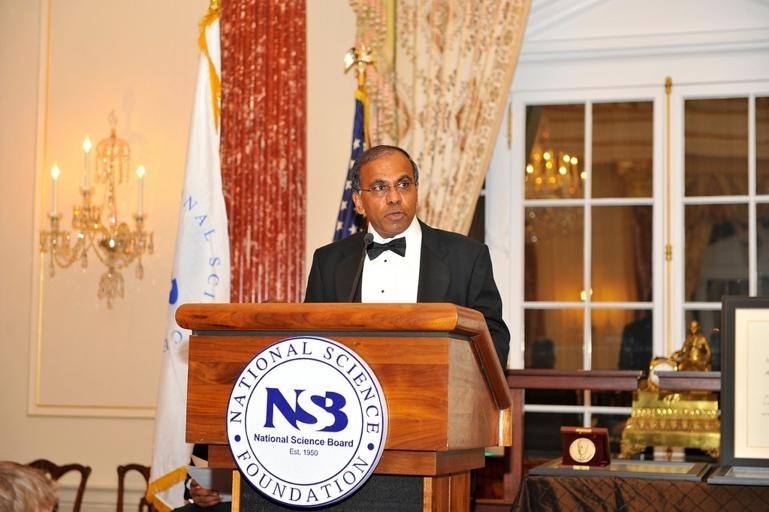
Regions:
[519,438,769,511]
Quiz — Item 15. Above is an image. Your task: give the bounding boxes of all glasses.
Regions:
[351,180,417,196]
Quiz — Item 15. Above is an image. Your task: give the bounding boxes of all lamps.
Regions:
[39,118,152,309]
[524,136,596,242]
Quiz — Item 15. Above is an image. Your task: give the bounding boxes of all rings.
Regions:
[197,496,201,502]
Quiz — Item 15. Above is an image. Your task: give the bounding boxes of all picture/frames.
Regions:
[720,298,769,473]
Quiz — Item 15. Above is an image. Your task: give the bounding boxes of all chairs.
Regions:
[117,464,152,512]
[21,457,91,511]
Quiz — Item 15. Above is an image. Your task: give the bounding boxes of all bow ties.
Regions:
[363,237,409,261]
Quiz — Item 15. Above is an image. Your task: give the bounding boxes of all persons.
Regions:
[1,460,59,512]
[170,443,233,512]
[302,144,510,512]
[671,319,712,372]
[615,313,651,381]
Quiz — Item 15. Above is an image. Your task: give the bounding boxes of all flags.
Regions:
[145,11,233,512]
[330,91,373,245]
